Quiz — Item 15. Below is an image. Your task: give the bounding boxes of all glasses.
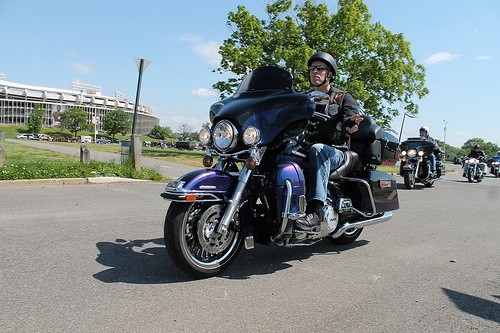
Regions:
[308,65,328,71]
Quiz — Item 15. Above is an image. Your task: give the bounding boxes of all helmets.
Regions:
[419,126,430,136]
[308,52,337,76]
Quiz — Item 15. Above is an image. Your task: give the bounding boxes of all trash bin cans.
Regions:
[120,140,131,165]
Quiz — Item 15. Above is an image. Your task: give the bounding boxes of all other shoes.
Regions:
[432,170,437,175]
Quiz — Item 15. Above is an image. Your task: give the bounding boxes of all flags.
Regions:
[406,110,417,118]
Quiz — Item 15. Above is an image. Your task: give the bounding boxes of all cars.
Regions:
[16,131,176,149]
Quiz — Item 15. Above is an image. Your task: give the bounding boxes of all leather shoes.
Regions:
[295,212,321,232]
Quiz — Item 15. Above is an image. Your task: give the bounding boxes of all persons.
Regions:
[53,105,63,121]
[288,52,363,232]
[463,145,485,177]
[461,154,468,168]
[420,127,440,175]
[489,151,500,173]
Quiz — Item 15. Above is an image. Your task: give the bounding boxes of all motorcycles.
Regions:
[398,136,446,190]
[158,63,403,280]
[490,154,500,178]
[462,155,488,183]
